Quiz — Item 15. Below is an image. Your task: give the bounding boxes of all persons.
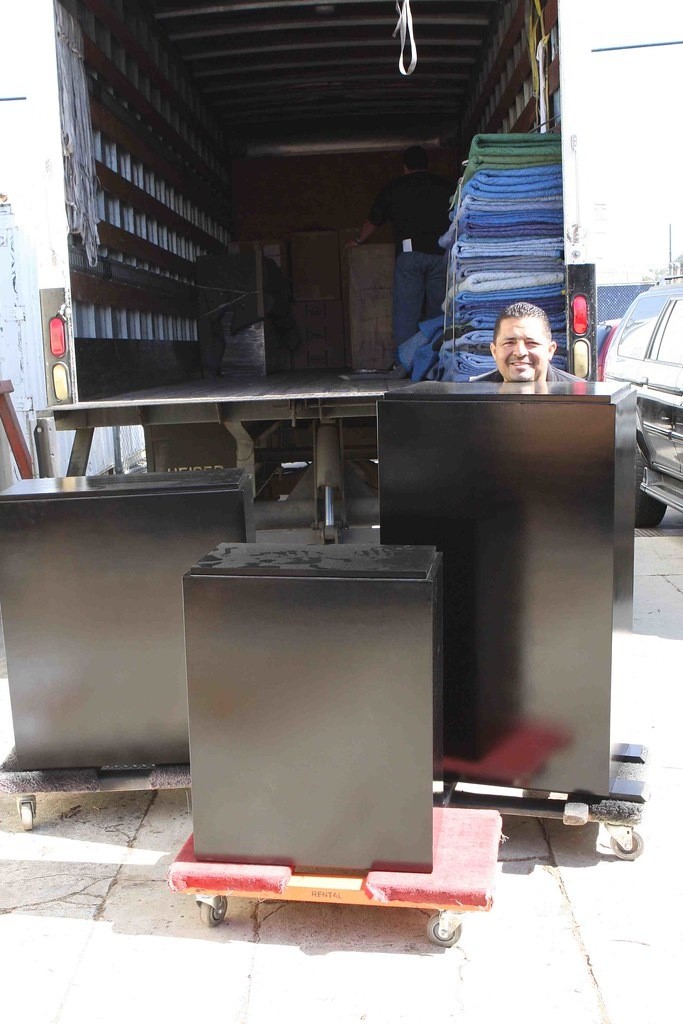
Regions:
[469,302,589,384]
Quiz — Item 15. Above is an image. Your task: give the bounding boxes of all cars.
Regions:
[603,282,683,529]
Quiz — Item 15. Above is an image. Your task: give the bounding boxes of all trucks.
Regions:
[36,0,598,546]
